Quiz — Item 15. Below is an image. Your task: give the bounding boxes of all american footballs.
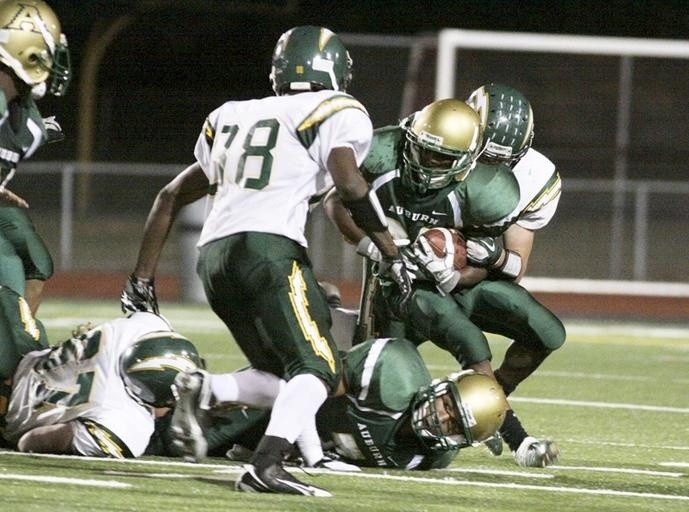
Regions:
[416,227,468,270]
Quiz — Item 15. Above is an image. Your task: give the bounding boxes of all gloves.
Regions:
[466,237,502,264]
[377,236,461,305]
[121,274,158,318]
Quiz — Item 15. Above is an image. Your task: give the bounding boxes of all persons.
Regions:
[323,97,567,468]
[0,0,71,318]
[143,339,508,470]
[120,25,416,498]
[351,81,562,345]
[0,286,207,458]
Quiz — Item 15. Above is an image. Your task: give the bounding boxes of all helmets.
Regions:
[465,83,534,168]
[399,99,481,192]
[119,331,204,407]
[0,0,66,99]
[409,371,507,449]
[269,26,353,96]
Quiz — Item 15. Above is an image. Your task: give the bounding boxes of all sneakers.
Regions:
[237,457,362,498]
[513,437,558,467]
[169,372,208,461]
